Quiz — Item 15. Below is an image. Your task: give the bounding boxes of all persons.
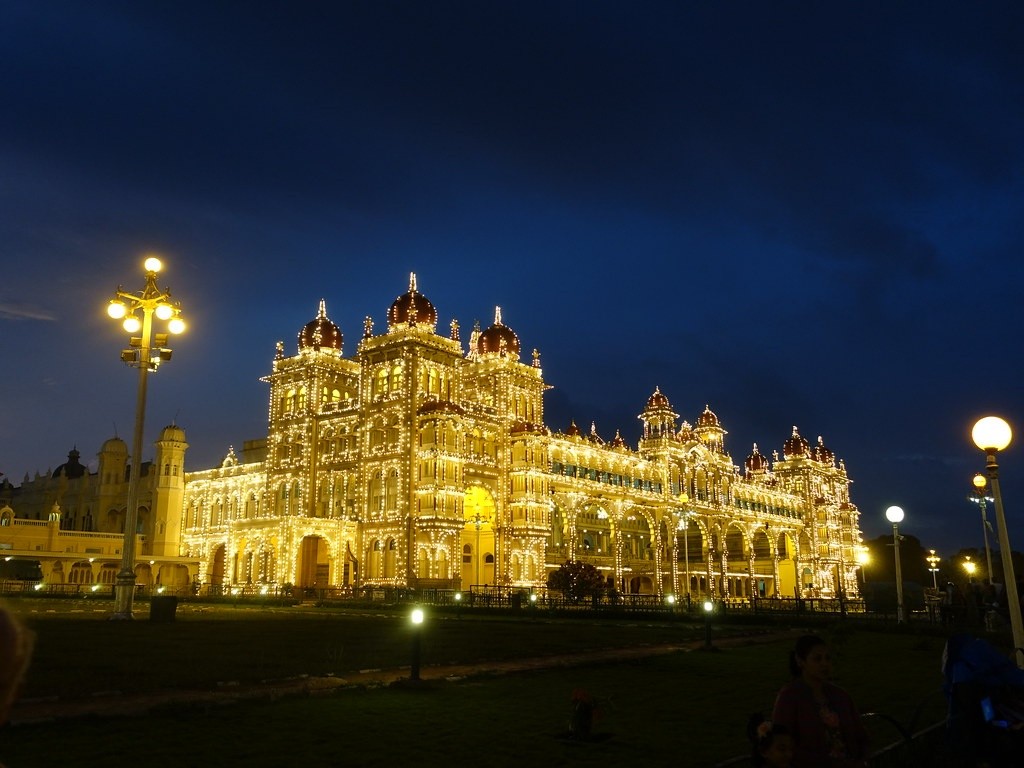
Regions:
[755,634,871,768]
